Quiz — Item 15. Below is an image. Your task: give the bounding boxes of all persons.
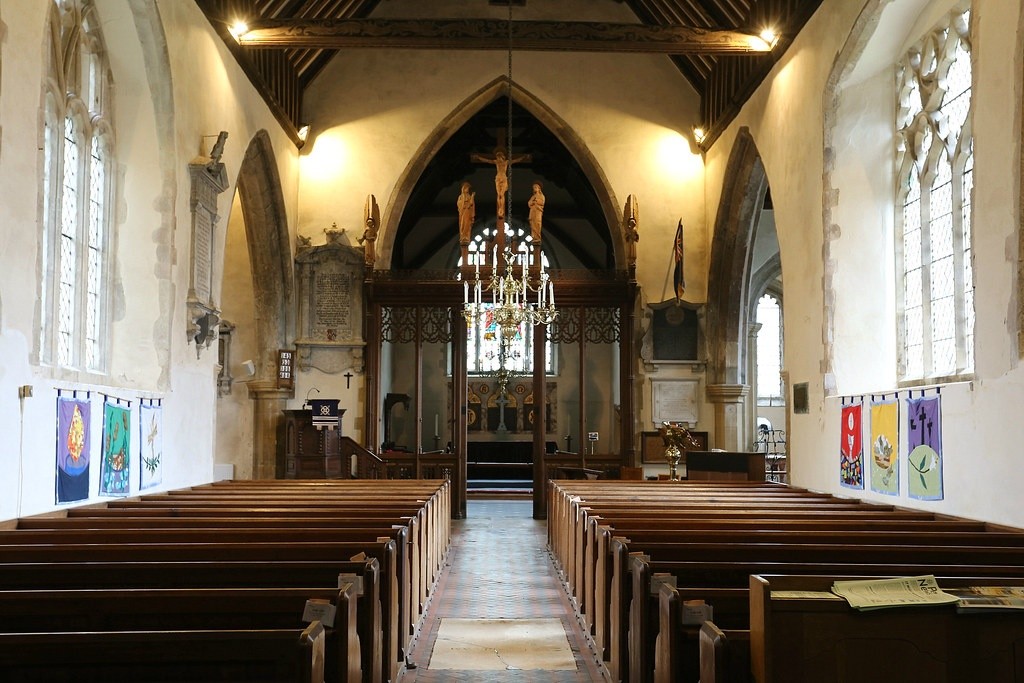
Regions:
[625,219,639,266]
[362,217,377,263]
[456,182,476,244]
[527,183,545,243]
[471,151,529,219]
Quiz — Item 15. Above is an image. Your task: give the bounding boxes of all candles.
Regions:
[435,414,438,436]
[460,244,560,350]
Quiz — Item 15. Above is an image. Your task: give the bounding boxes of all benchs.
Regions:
[548,476,1024,683]
[0,478,450,683]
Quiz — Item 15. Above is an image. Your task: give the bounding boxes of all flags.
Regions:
[673,226,686,303]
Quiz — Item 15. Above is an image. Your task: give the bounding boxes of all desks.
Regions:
[686,451,767,481]
[641,431,708,464]
